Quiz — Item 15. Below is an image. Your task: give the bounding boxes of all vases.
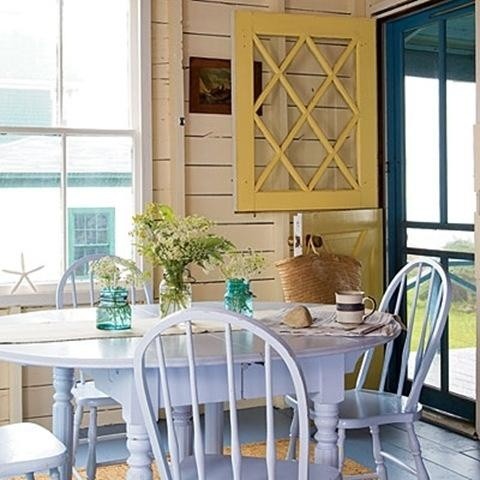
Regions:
[224,278,254,320]
[96,287,132,330]
[159,269,192,318]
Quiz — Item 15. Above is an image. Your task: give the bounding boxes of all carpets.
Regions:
[0,436,378,480]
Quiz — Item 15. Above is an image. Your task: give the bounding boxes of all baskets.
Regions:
[274,232,363,305]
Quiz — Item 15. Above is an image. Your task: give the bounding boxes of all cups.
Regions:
[334,290,375,323]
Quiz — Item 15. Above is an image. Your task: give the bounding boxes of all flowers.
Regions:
[87,256,150,326]
[129,201,236,315]
[219,248,267,312]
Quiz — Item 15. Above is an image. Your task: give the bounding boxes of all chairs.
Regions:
[285,257,452,480]
[133,306,342,480]
[0,422,68,480]
[56,254,154,480]
